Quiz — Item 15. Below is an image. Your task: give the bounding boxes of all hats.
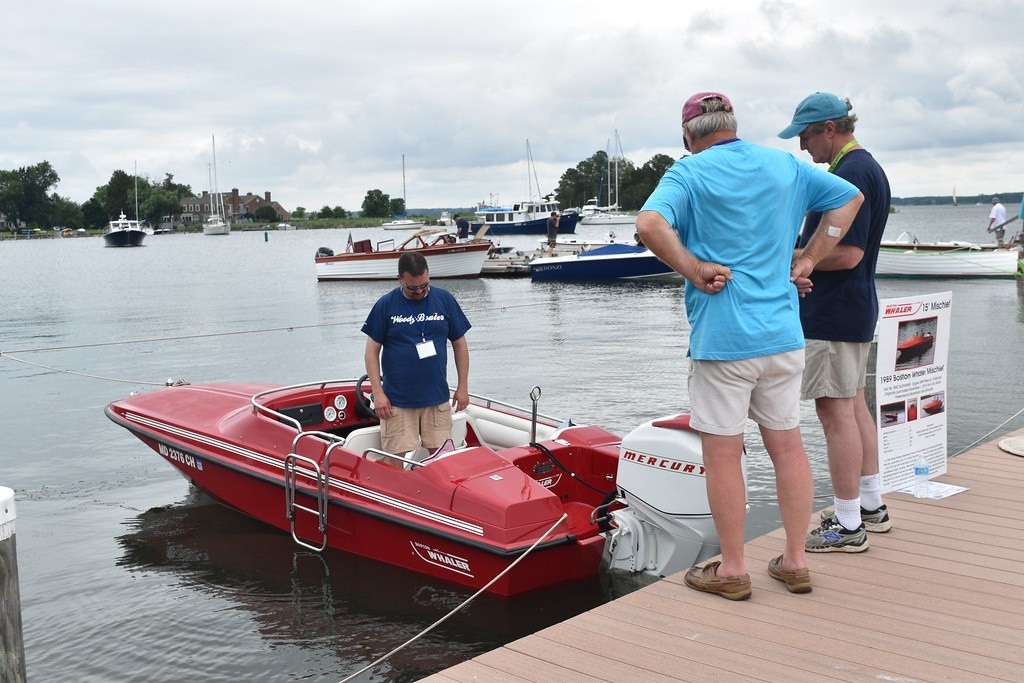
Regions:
[777,92,848,139]
[991,196,1000,203]
[682,92,733,152]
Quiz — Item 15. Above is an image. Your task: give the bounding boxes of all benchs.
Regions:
[342,411,468,464]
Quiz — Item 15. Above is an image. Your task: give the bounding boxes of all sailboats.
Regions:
[470,139,581,236]
[581,129,638,225]
[202,134,230,236]
[381,154,426,230]
[128,161,155,235]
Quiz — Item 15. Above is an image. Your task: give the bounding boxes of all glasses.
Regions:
[401,277,429,290]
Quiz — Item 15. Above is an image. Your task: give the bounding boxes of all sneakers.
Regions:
[684,560,752,600]
[768,554,812,593]
[805,515,869,552]
[820,504,892,532]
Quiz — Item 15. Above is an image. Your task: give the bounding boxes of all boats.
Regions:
[541,240,636,254]
[60,227,86,238]
[885,413,897,419]
[154,227,171,235]
[560,197,598,218]
[103,210,149,247]
[277,224,297,230]
[873,232,1020,277]
[313,227,493,280]
[437,211,457,228]
[528,242,679,282]
[104,377,752,599]
[923,398,942,412]
[897,333,933,353]
[481,246,533,277]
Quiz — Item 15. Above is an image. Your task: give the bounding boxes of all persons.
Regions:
[548,211,560,258]
[635,93,864,601]
[362,251,473,469]
[779,93,891,553]
[454,214,469,243]
[988,196,1006,249]
